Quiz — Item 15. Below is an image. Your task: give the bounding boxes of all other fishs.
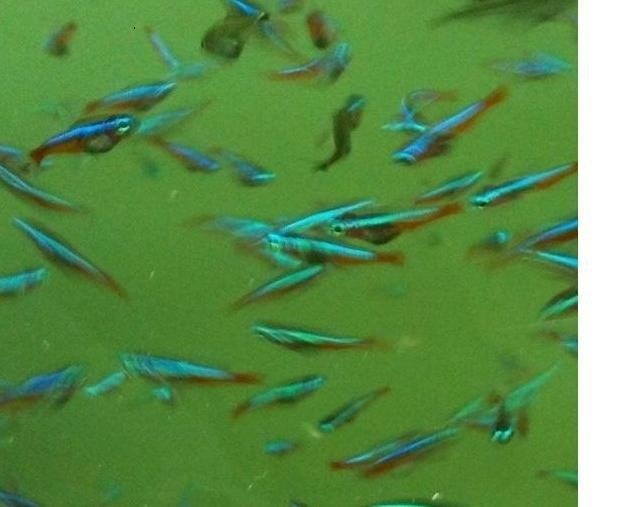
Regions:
[0,0,577,506]
